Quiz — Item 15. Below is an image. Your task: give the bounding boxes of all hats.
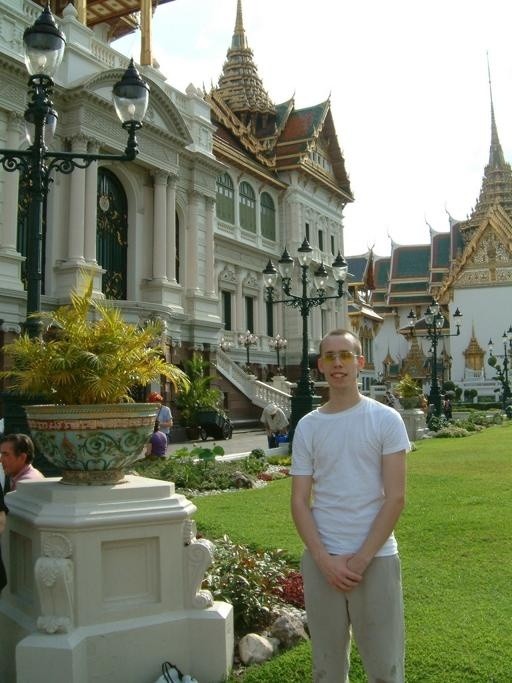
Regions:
[266,404,277,416]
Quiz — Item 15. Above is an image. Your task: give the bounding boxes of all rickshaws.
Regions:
[193,402,234,441]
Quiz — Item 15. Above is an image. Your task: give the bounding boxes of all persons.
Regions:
[444,399,453,418]
[0,483,8,593]
[416,393,428,409]
[259,403,289,449]
[146,392,173,439]
[287,329,413,683]
[0,432,46,490]
[380,390,396,409]
[145,421,168,458]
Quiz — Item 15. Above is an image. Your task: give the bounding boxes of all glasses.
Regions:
[324,352,359,365]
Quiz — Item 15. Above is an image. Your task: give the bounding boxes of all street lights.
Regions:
[487,323,512,410]
[0,1,150,480]
[407,297,463,418]
[433,349,454,386]
[261,236,348,456]
